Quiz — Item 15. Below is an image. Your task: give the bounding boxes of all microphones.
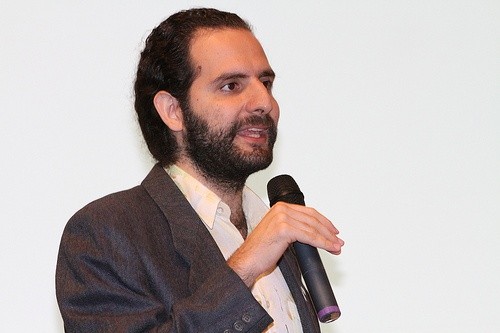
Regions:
[267,175,341,323]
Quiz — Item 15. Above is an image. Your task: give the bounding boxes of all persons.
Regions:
[54,7,346,333]
